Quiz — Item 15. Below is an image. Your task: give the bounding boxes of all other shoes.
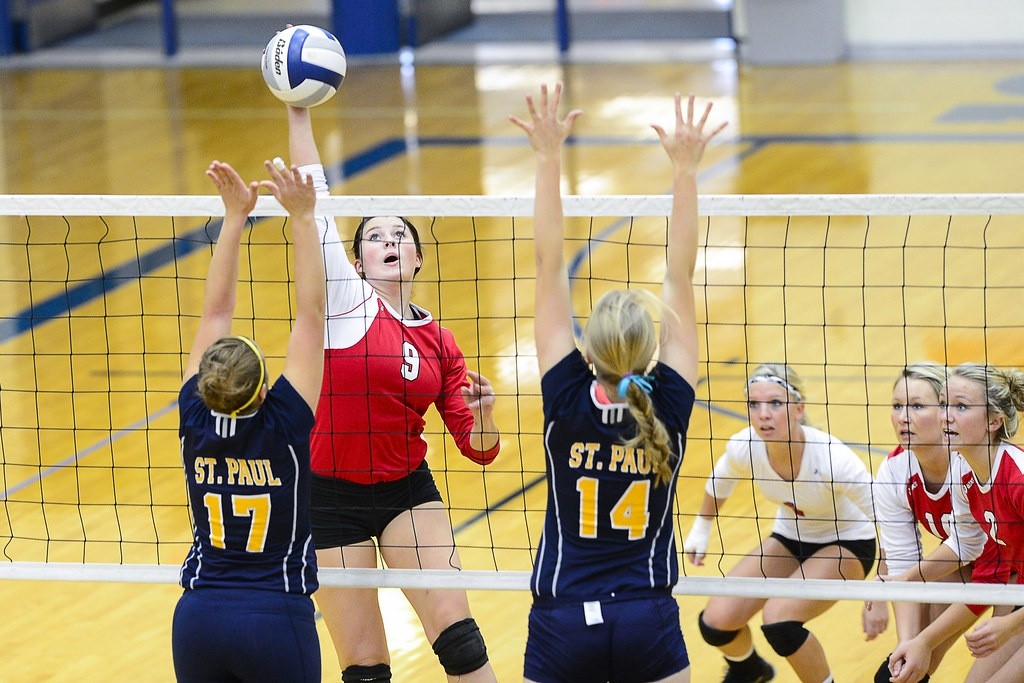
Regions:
[721,657,774,683]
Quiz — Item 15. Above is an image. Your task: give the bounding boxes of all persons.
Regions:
[257,25,503,682]
[684,361,888,683]
[501,85,728,682]
[168,155,325,682]
[888,364,1024,683]
[869,364,988,683]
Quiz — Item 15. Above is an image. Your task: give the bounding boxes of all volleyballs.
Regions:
[258,22,348,109]
